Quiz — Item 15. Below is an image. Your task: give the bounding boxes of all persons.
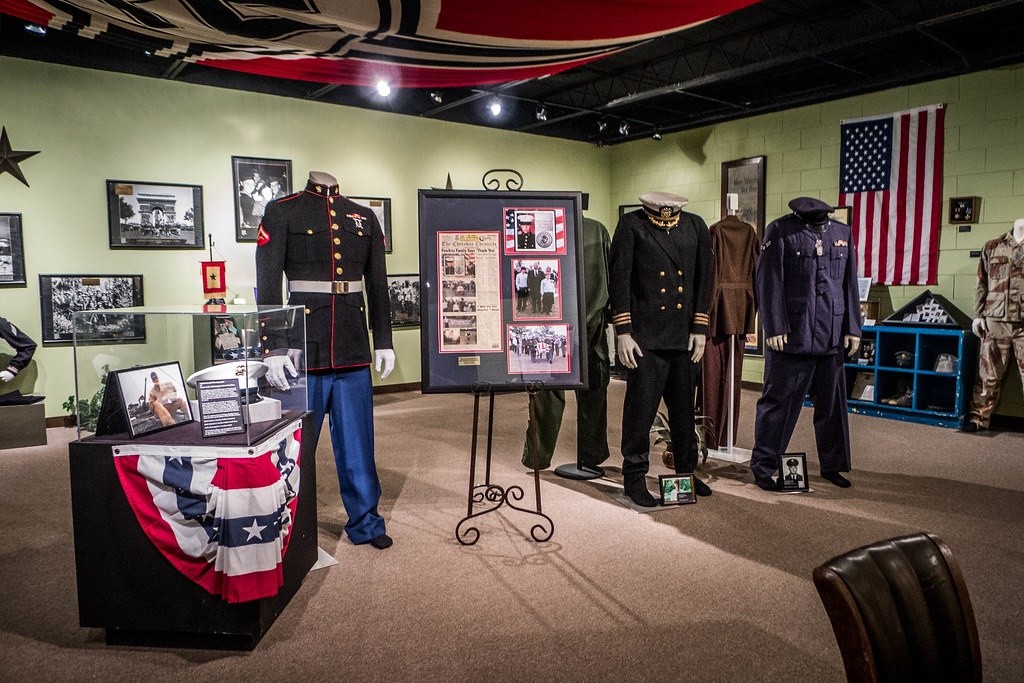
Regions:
[0,316,37,384]
[439,254,478,346]
[750,196,868,490]
[961,218,1023,433]
[254,169,401,551]
[787,459,802,483]
[49,276,137,336]
[670,478,681,502]
[148,372,190,421]
[709,192,760,450]
[613,191,714,505]
[387,276,418,324]
[519,210,613,468]
[507,215,569,364]
[240,173,286,228]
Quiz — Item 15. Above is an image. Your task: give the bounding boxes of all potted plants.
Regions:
[652,407,718,470]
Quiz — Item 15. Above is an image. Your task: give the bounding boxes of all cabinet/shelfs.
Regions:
[801,322,979,429]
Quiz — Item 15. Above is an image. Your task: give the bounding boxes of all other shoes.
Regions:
[889,387,913,407]
[878,384,906,404]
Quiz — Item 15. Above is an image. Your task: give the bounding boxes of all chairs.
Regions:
[812,531,987,683]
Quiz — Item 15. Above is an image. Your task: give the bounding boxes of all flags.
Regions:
[839,104,943,289]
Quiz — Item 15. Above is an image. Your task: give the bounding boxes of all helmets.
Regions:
[932,354,958,372]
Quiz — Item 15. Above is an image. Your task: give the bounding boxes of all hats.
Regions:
[639,191,688,230]
[893,350,913,367]
[151,372,157,380]
[186,360,270,406]
[788,197,835,234]
[786,459,799,466]
[517,214,534,225]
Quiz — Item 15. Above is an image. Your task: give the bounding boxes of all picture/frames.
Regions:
[384,274,422,328]
[658,473,697,506]
[106,178,206,250]
[344,196,393,254]
[826,206,852,226]
[0,211,26,286]
[114,358,194,440]
[418,188,592,395]
[777,452,810,492]
[232,155,294,242]
[38,273,146,343]
[947,196,975,224]
